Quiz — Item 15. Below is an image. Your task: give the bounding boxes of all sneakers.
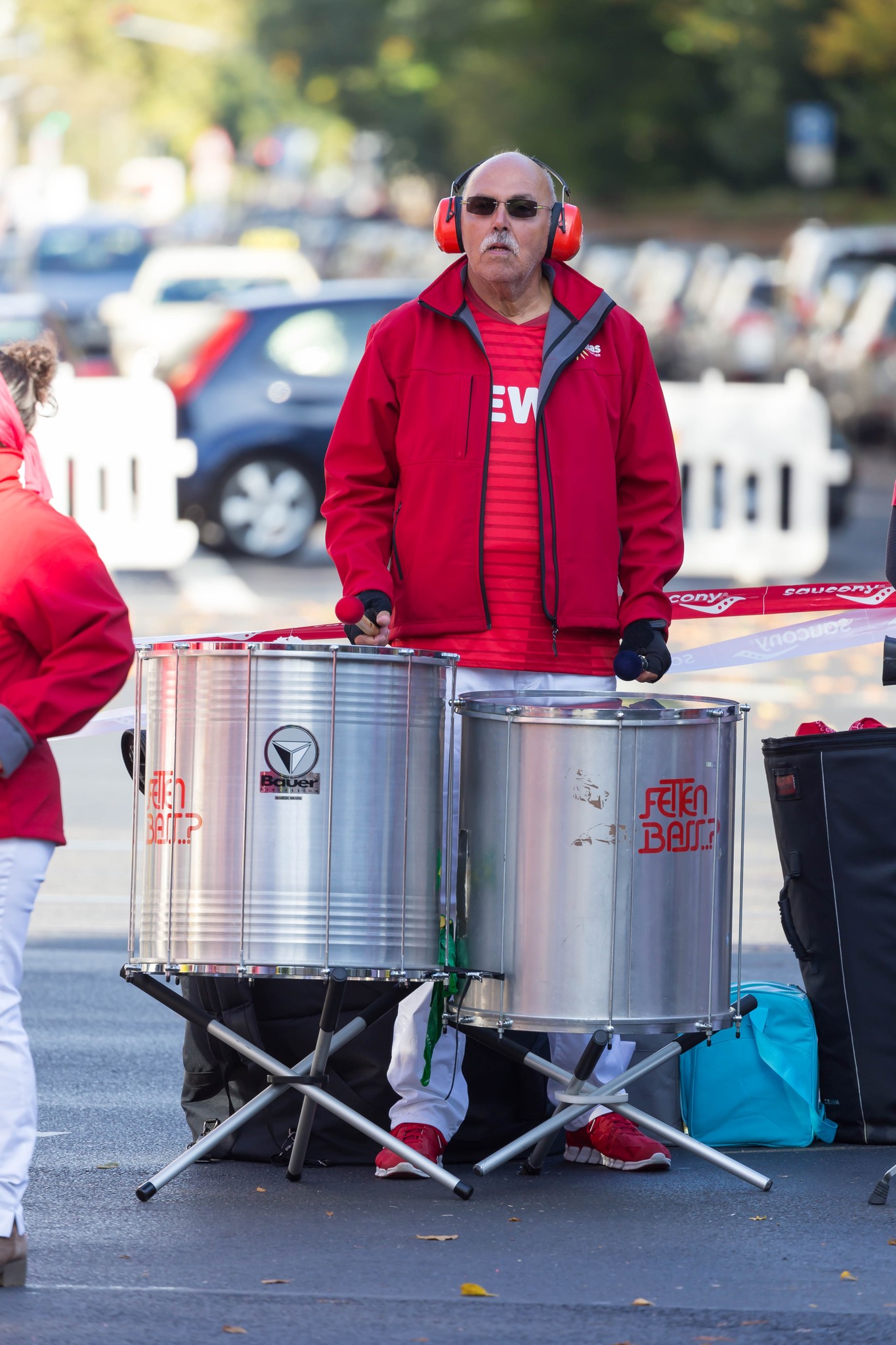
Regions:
[564,1112,670,1169]
[376,1122,448,1178]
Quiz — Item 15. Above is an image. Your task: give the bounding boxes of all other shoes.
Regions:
[0,1221,27,1287]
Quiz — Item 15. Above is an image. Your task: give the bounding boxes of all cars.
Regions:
[0,201,467,382]
[160,279,858,561]
[569,213,896,446]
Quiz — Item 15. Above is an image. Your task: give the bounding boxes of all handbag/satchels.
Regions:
[177,970,560,1161]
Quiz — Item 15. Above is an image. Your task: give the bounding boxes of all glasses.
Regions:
[462,195,552,218]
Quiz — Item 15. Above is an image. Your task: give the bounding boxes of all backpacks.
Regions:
[685,980,836,1149]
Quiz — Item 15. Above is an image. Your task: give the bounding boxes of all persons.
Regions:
[0,344,141,1286]
[319,152,683,1178]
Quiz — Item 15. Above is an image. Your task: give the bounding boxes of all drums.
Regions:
[467,685,743,1040]
[132,633,458,997]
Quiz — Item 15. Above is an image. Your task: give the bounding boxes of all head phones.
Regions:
[432,154,585,262]
